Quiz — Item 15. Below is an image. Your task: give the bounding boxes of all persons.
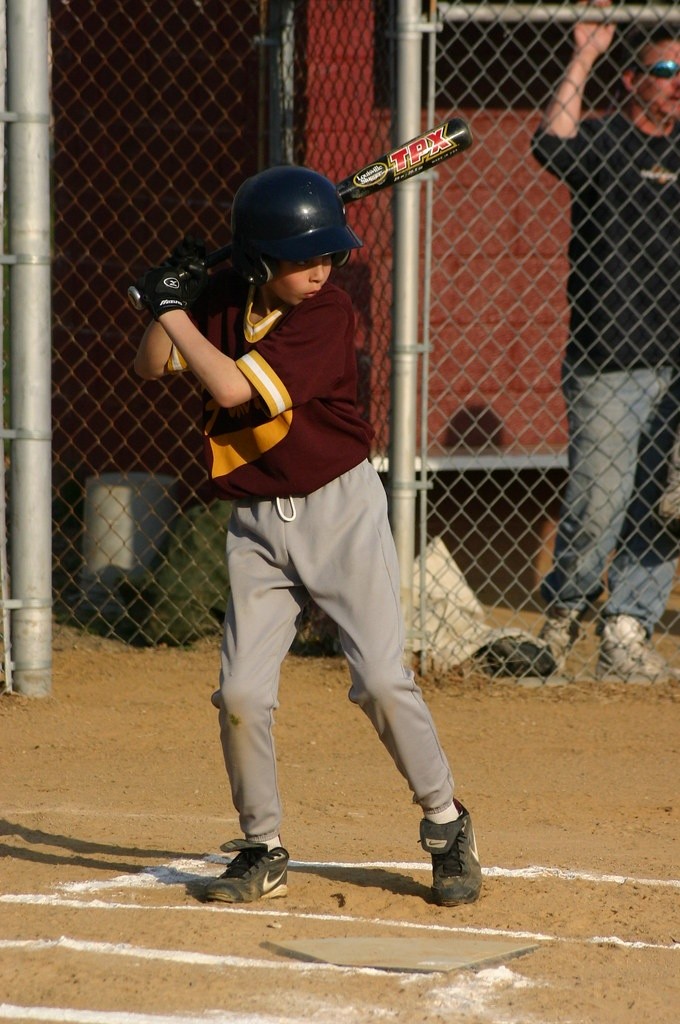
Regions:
[530,0,680,687]
[134,164,484,907]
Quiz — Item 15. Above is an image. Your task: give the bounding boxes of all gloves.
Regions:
[137,260,188,321]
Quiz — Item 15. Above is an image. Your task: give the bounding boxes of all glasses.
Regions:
[637,60,680,79]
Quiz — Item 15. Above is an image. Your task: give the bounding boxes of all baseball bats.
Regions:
[127,119,473,312]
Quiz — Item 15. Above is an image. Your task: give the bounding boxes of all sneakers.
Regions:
[206,839,291,904]
[536,617,580,666]
[418,796,483,907]
[595,623,667,686]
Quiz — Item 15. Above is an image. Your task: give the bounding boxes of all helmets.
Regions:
[228,165,365,285]
